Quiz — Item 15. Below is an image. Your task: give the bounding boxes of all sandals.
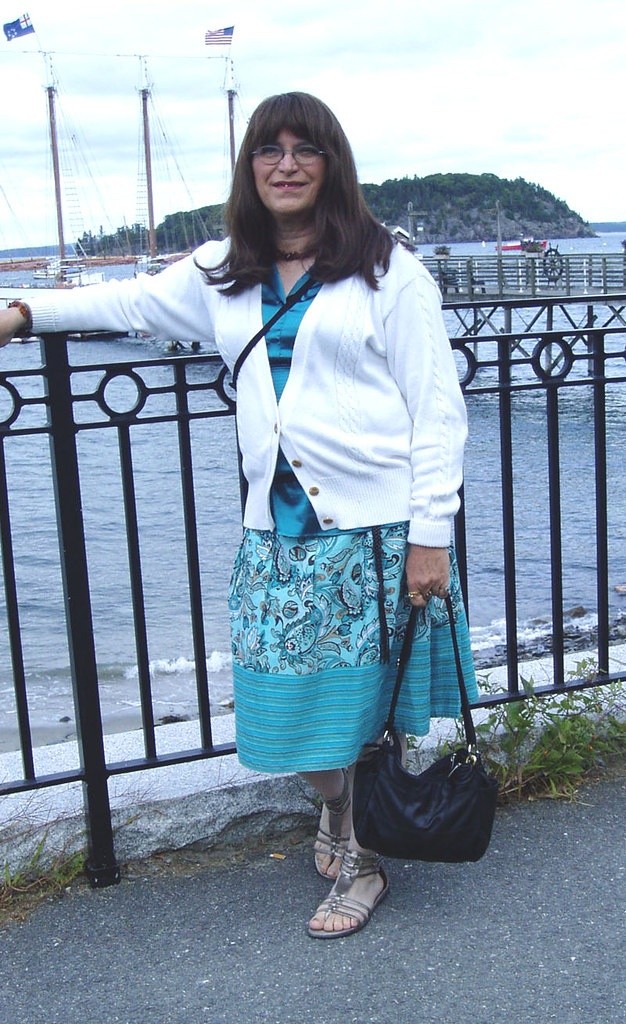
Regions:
[307,851,391,939]
[313,768,352,881]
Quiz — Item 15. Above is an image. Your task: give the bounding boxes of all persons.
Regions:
[0,93,479,938]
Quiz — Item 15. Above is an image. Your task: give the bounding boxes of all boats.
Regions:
[29,253,192,289]
[495,239,547,253]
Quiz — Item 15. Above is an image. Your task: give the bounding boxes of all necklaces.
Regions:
[276,247,318,261]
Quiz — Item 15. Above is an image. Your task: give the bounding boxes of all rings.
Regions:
[408,591,421,598]
[423,591,432,598]
[441,587,449,593]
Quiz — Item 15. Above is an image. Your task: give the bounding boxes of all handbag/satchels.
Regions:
[353,592,496,863]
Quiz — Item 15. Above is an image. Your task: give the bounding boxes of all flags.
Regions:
[3,13,36,41]
[206,26,235,45]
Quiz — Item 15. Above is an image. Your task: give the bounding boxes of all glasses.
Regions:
[251,145,326,165]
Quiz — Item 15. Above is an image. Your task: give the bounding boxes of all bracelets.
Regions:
[8,301,32,338]
